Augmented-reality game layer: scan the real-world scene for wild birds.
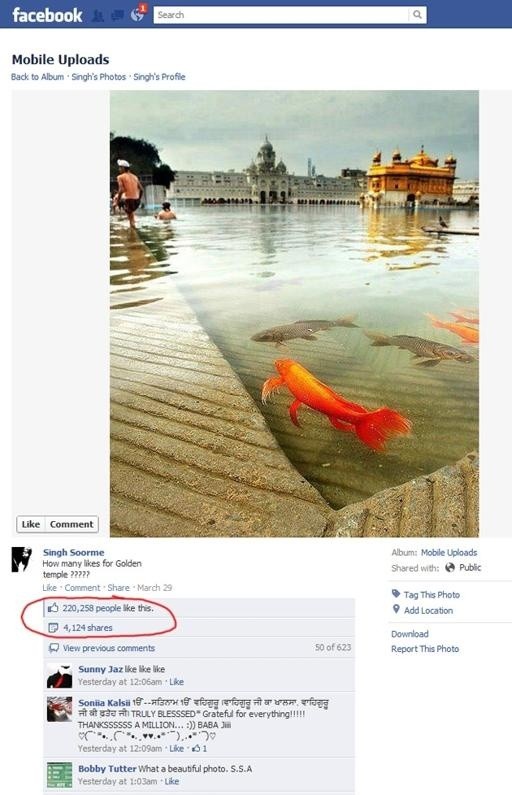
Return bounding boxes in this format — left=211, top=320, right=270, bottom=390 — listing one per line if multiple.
left=437, top=214, right=448, bottom=228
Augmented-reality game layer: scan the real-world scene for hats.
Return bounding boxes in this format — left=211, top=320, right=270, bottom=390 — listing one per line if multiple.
left=117, top=159, right=130, bottom=167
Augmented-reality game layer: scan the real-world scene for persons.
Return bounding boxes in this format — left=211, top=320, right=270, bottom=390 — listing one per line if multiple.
left=111, top=159, right=144, bottom=230
left=155, top=202, right=176, bottom=219
